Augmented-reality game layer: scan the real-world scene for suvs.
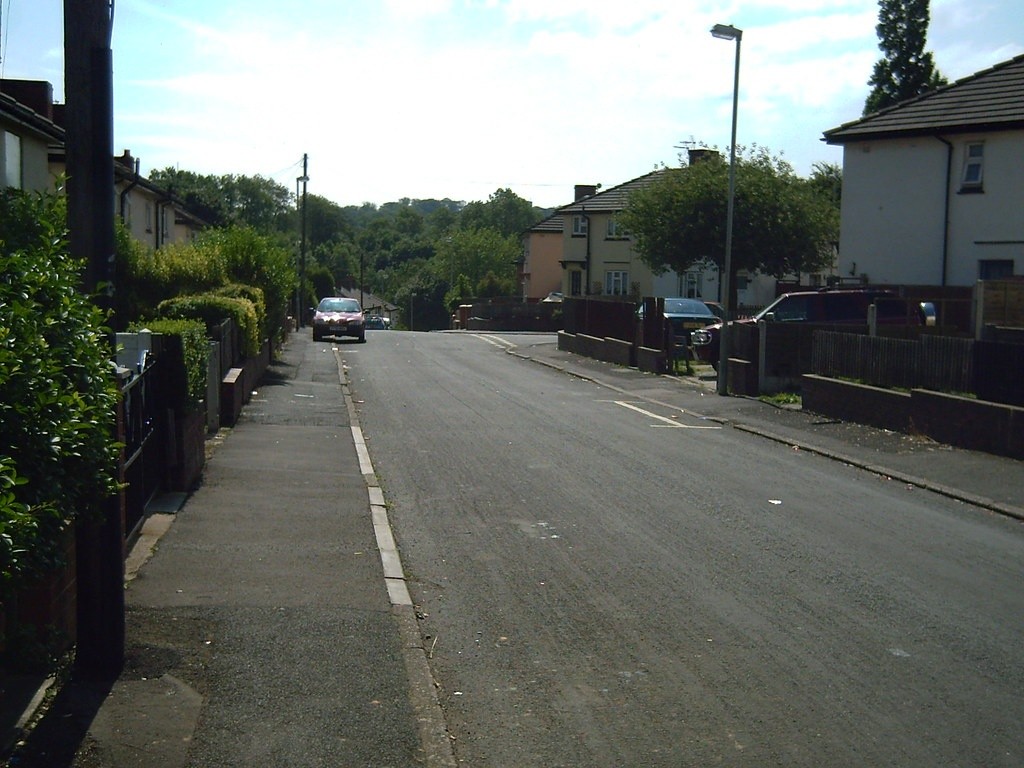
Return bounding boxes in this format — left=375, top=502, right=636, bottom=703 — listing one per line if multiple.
left=692, top=282, right=938, bottom=389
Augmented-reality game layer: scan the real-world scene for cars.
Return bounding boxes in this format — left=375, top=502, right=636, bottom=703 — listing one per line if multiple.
left=364, top=313, right=391, bottom=330
left=308, top=296, right=371, bottom=344
left=635, top=297, right=722, bottom=344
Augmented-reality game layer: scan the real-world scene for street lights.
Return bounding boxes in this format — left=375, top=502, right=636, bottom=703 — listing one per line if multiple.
left=295, top=175, right=310, bottom=333
left=708, top=22, right=745, bottom=395
